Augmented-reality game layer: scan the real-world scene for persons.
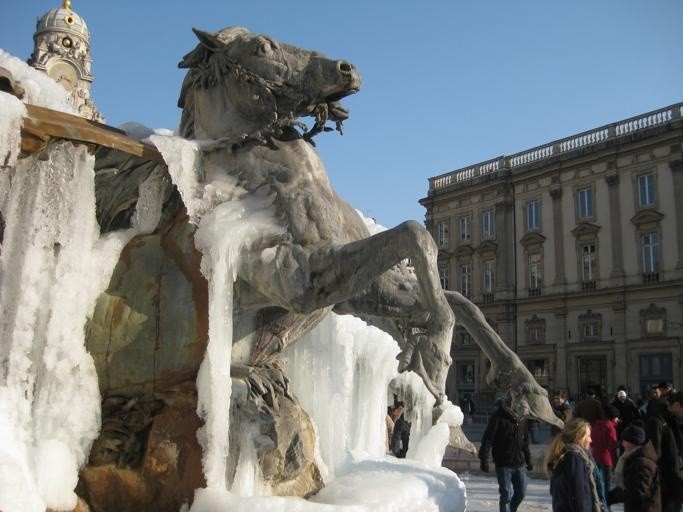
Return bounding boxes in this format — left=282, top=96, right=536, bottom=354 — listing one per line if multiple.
left=40, top=35, right=63, bottom=64
left=67, top=40, right=82, bottom=60
left=503, top=383, right=517, bottom=410
left=387, top=405, right=394, bottom=452
left=477, top=399, right=534, bottom=512
left=390, top=399, right=411, bottom=460
left=546, top=380, right=682, bottom=512
left=391, top=407, right=398, bottom=423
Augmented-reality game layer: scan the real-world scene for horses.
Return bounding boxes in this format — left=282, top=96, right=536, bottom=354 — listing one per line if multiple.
left=95, top=26, right=564, bottom=432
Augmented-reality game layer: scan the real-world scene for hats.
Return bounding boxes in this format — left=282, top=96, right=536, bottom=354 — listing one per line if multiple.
left=617, top=389, right=628, bottom=398
left=619, top=425, right=646, bottom=446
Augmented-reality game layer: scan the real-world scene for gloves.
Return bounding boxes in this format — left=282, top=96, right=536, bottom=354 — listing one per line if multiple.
left=480, top=460, right=489, bottom=473
left=527, top=460, right=533, bottom=471
